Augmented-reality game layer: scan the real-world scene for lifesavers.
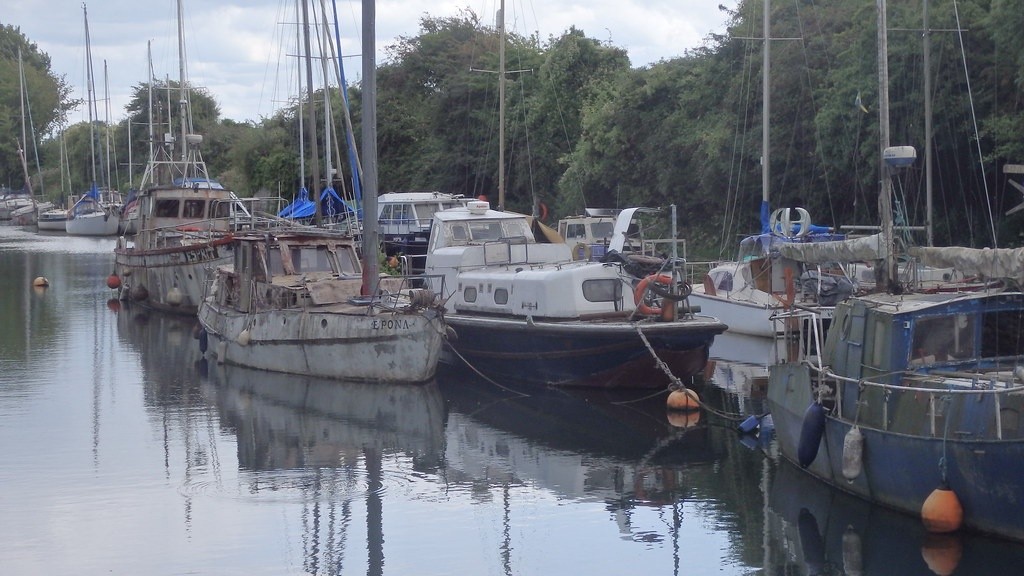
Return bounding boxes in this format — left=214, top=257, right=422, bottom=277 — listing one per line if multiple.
left=633, top=275, right=675, bottom=315
left=573, top=243, right=590, bottom=261
left=175, top=226, right=202, bottom=231
left=539, top=203, right=547, bottom=220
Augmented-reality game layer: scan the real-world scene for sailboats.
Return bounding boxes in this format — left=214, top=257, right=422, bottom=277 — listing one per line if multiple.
left=766, top=0, right=1024, bottom=543
left=0, top=0, right=941, bottom=385
